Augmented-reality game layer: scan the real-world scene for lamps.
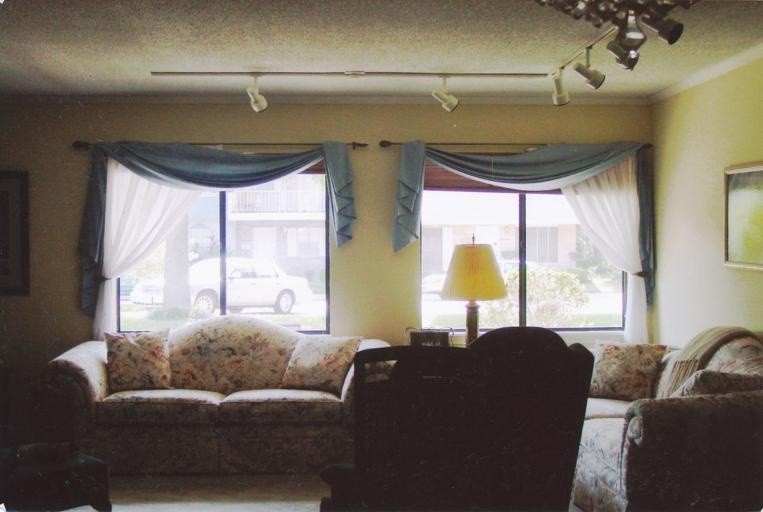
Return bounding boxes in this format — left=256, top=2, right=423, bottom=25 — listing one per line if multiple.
left=440, top=233, right=508, bottom=347
left=151, top=1, right=695, bottom=112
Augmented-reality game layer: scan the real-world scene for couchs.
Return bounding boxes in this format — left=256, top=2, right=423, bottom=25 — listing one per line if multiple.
left=42, top=314, right=394, bottom=478
left=574, top=325, right=763, bottom=512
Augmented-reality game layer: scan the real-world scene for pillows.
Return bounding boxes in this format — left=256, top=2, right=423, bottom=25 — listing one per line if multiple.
left=587, top=338, right=667, bottom=400
left=103, top=322, right=361, bottom=394
left=666, top=369, right=763, bottom=396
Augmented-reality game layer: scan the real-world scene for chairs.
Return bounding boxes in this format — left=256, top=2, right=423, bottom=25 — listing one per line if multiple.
left=319, top=328, right=594, bottom=512
left=0, top=362, right=114, bottom=512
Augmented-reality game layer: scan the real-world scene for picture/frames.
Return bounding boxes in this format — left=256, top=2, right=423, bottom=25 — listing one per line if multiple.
left=722, top=161, right=763, bottom=272
left=0, top=170, right=29, bottom=296
left=404, top=326, right=454, bottom=347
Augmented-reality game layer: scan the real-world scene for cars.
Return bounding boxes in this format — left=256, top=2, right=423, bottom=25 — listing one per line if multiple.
left=423, top=259, right=533, bottom=295
left=132, top=259, right=310, bottom=315
